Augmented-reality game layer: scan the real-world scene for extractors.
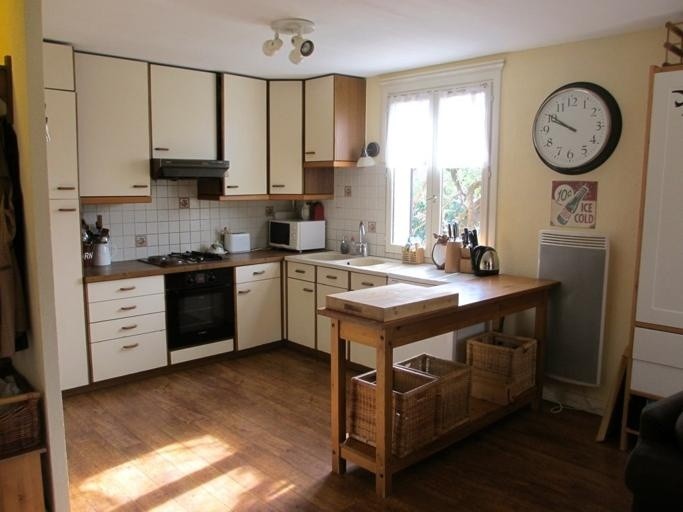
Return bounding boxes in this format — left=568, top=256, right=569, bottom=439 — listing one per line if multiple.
left=150, top=158, right=230, bottom=181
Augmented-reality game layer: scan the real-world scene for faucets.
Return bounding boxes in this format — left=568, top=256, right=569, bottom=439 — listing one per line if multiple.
left=357, top=220, right=366, bottom=255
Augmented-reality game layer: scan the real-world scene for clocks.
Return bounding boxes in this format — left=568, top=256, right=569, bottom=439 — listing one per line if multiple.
left=532, top=82, right=620, bottom=175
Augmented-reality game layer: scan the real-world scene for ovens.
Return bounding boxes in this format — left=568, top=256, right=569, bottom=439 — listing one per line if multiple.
left=162, top=266, right=233, bottom=351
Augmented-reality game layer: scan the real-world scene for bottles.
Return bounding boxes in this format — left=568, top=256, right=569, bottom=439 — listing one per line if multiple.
left=82, top=243, right=94, bottom=268
left=556, top=186, right=588, bottom=225
left=340, top=221, right=365, bottom=256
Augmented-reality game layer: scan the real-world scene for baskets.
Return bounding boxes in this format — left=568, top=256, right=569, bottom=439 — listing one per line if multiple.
left=349, top=367, right=435, bottom=458
left=466, top=331, right=537, bottom=406
left=1, top=359, right=41, bottom=457
left=393, top=353, right=469, bottom=432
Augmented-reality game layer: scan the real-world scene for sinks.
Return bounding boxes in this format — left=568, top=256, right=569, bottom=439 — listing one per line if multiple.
left=342, top=257, right=386, bottom=268
left=300, top=253, right=355, bottom=263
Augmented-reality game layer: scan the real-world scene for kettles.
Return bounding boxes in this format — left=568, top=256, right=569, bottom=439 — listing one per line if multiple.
left=468, top=244, right=500, bottom=277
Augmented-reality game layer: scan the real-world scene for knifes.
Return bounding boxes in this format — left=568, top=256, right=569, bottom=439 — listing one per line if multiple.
left=448, top=222, right=478, bottom=246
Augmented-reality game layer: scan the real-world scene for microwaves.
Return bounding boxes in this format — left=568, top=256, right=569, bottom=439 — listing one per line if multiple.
left=268, top=217, right=325, bottom=254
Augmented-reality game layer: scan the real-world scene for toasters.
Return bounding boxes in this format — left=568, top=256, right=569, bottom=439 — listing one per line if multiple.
left=223, top=231, right=250, bottom=253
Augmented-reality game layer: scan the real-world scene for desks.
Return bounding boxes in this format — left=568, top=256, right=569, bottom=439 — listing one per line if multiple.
left=316, top=272, right=564, bottom=500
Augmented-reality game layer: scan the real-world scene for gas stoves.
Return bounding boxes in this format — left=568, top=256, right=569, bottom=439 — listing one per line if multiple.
left=138, top=250, right=223, bottom=268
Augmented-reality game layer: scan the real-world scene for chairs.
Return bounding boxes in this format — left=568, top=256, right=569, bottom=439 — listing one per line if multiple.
left=622, top=393, right=682, bottom=512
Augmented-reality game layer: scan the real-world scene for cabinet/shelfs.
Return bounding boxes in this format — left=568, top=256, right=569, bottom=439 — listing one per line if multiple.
left=287, top=258, right=353, bottom=363
left=230, top=250, right=283, bottom=353
left=628, top=65, right=681, bottom=399
left=31, top=38, right=91, bottom=400
left=268, top=73, right=335, bottom=201
left=66, top=48, right=151, bottom=207
left=148, top=62, right=218, bottom=179
left=0, top=446, right=49, bottom=512
left=83, top=258, right=167, bottom=390
left=304, top=76, right=366, bottom=168
left=345, top=274, right=386, bottom=371
left=198, top=72, right=269, bottom=202
left=392, top=275, right=458, bottom=374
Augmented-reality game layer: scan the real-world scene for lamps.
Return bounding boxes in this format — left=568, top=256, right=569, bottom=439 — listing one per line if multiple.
left=356, top=142, right=380, bottom=167
left=262, top=18, right=316, bottom=65
